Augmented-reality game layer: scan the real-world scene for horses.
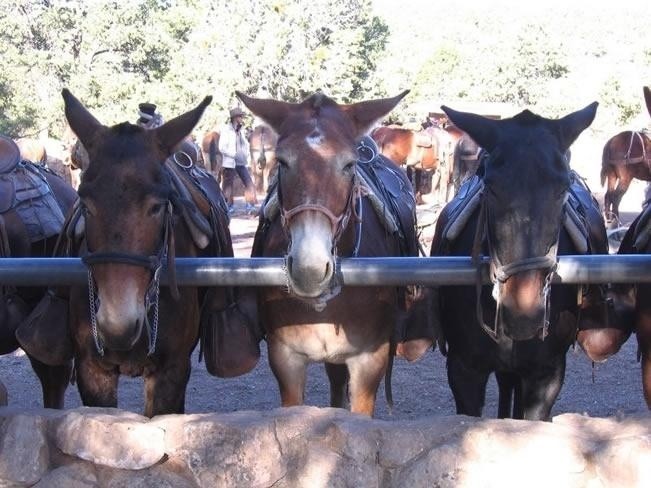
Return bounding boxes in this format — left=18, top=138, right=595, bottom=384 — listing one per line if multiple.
left=200, top=120, right=276, bottom=197
left=3, top=144, right=91, bottom=420
left=428, top=101, right=607, bottom=422
left=371, top=109, right=501, bottom=198
left=58, top=91, right=231, bottom=417
left=237, top=88, right=422, bottom=416
left=601, top=124, right=651, bottom=221
left=616, top=88, right=650, bottom=421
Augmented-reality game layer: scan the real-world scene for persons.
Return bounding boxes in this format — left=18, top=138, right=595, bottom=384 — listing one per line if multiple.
left=373, top=113, right=461, bottom=211
left=215, top=105, right=261, bottom=216
